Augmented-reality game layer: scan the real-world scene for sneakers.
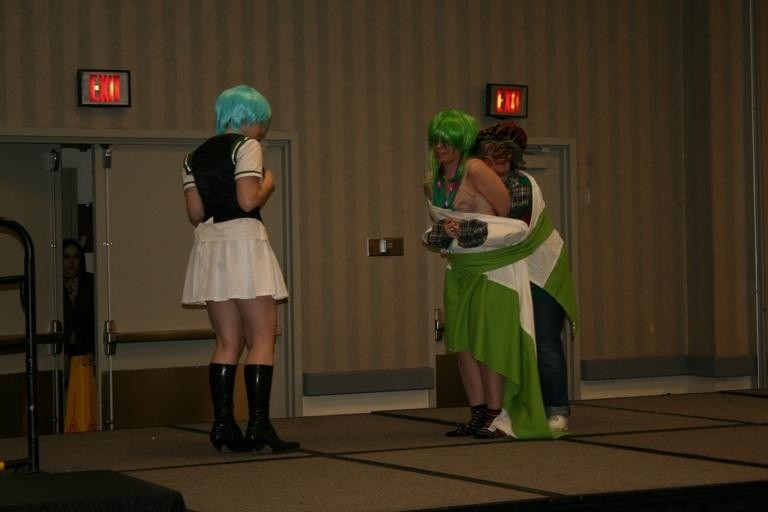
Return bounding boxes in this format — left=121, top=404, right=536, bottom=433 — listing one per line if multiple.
left=546, top=415, right=569, bottom=432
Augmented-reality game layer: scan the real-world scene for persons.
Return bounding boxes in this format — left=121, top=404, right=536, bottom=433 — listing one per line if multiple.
left=421, top=120, right=580, bottom=436
left=60, top=236, right=96, bottom=433
left=421, top=108, right=513, bottom=440
left=182, top=84, right=303, bottom=452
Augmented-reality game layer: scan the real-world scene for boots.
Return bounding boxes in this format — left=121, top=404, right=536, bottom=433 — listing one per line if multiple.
left=210, top=364, right=253, bottom=454
left=245, top=364, right=299, bottom=454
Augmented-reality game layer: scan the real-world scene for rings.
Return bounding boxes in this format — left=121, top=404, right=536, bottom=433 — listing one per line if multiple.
left=450, top=227, right=455, bottom=232
